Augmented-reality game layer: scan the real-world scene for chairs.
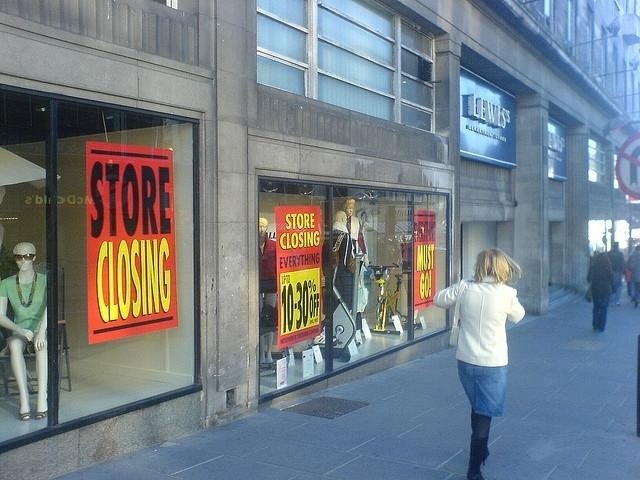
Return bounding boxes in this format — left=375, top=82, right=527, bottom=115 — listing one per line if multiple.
left=0, top=269, right=71, bottom=393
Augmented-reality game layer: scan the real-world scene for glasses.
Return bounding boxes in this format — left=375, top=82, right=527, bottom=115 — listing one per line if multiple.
left=14, top=253, right=36, bottom=262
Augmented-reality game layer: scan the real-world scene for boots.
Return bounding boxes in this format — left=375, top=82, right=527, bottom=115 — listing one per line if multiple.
left=466, top=434, right=489, bottom=480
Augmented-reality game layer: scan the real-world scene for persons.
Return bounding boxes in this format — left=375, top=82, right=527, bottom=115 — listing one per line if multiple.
left=0, top=241, right=49, bottom=422
left=432, top=246, right=528, bottom=480
left=624, top=237, right=636, bottom=261
left=334, top=211, right=359, bottom=360
left=344, top=197, right=368, bottom=347
left=589, top=241, right=615, bottom=333
left=258, top=217, right=275, bottom=366
left=608, top=242, right=624, bottom=307
left=627, top=244, right=640, bottom=308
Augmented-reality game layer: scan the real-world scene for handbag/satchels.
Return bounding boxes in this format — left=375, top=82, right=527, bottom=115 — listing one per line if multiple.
left=450, top=280, right=467, bottom=348
left=332, top=232, right=344, bottom=267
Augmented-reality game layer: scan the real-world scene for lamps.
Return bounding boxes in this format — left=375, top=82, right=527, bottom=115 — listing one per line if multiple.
left=592, top=55, right=640, bottom=84
left=565, top=14, right=621, bottom=55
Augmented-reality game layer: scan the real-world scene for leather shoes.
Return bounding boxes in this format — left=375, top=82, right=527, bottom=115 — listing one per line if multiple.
left=34, top=404, right=48, bottom=419
left=19, top=409, right=31, bottom=420
